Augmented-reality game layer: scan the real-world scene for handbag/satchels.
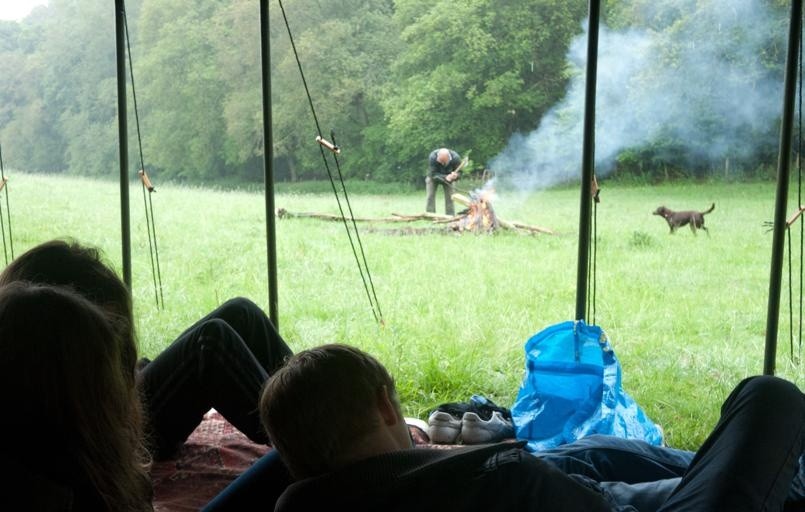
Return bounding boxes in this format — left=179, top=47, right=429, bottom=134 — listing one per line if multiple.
left=509, top=320, right=665, bottom=454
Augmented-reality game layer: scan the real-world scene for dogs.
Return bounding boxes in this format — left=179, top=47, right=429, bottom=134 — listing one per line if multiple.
left=652, top=202, right=716, bottom=239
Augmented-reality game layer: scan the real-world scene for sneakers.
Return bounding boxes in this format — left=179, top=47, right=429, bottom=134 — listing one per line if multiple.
left=428, top=410, right=461, bottom=443
left=462, top=411, right=515, bottom=444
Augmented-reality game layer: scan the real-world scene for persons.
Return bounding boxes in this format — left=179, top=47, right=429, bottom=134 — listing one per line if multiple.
left=1, top=284, right=154, bottom=512
left=0, top=236, right=138, bottom=404
left=262, top=337, right=804, bottom=511
left=423, top=147, right=466, bottom=215
left=139, top=292, right=296, bottom=449
